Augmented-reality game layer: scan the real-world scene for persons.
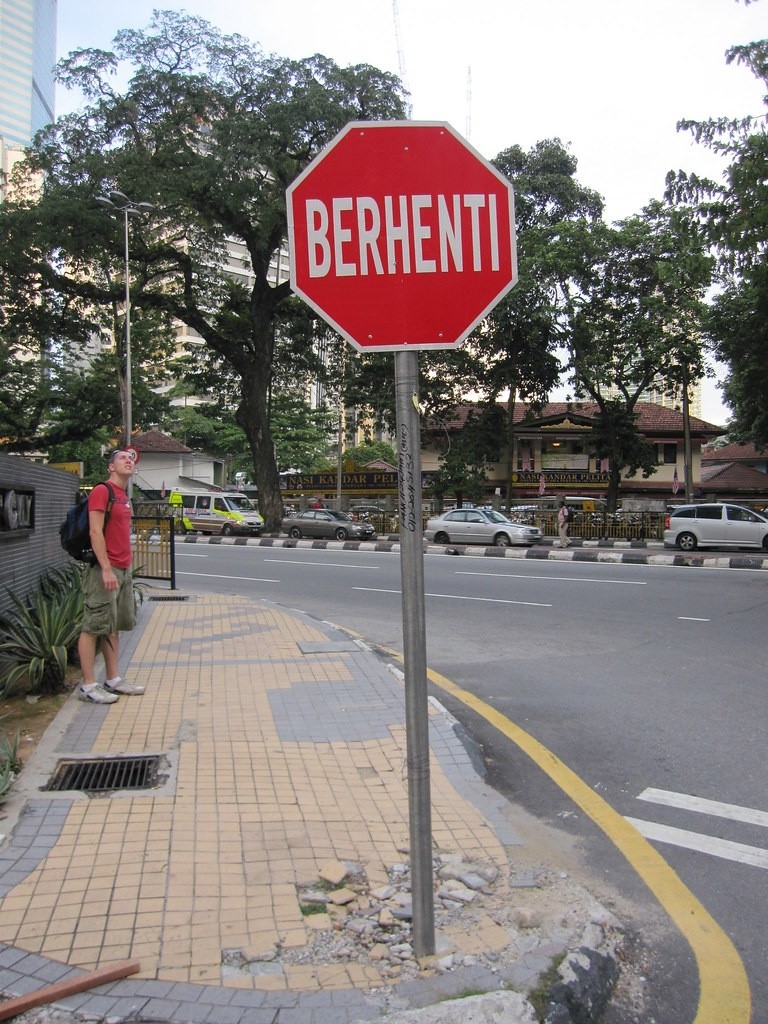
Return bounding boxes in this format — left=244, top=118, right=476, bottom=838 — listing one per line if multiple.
left=76, top=450, right=145, bottom=704
left=140, top=506, right=168, bottom=515
left=389, top=515, right=399, bottom=532
left=760, top=503, right=768, bottom=512
left=314, top=499, right=324, bottom=509
left=558, top=501, right=572, bottom=548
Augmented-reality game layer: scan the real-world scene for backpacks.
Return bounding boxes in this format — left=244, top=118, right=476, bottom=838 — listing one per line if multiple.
left=59, top=481, right=116, bottom=568
left=563, top=507, right=574, bottom=522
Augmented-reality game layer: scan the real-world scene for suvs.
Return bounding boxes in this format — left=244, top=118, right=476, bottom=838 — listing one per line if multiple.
left=662, top=502, right=768, bottom=552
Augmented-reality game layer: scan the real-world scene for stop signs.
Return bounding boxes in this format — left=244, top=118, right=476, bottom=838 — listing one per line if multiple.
left=285, top=118, right=519, bottom=353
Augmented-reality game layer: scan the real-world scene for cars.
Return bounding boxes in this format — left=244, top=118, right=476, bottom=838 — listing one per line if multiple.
left=442, top=501, right=539, bottom=525
left=346, top=506, right=386, bottom=524
left=424, top=508, right=543, bottom=547
left=280, top=508, right=377, bottom=542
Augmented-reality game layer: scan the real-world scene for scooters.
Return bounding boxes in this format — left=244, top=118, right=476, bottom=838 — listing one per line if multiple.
left=584, top=506, right=650, bottom=542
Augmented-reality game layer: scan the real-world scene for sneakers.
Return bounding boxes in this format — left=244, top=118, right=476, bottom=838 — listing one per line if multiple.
left=78, top=684, right=119, bottom=704
left=103, top=679, right=145, bottom=695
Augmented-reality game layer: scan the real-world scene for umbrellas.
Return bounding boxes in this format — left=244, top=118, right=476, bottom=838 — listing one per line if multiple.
left=161, top=483, right=165, bottom=497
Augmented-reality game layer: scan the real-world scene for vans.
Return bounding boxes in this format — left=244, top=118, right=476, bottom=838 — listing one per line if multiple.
left=537, top=496, right=606, bottom=511
left=166, top=486, right=266, bottom=537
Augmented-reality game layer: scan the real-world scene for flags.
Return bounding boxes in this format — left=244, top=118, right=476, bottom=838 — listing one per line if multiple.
left=673, top=469, right=680, bottom=494
left=540, top=475, right=545, bottom=495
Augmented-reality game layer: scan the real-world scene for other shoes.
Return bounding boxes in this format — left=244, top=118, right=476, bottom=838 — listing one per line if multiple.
left=566, top=541, right=573, bottom=547
left=557, top=546, right=567, bottom=549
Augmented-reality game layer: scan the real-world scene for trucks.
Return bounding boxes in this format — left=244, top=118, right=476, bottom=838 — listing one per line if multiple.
left=614, top=498, right=664, bottom=524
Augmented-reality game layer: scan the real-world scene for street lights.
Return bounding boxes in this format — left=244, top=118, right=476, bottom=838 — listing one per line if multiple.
left=93, top=189, right=157, bottom=514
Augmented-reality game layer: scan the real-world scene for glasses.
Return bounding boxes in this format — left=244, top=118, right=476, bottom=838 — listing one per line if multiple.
left=111, top=450, right=120, bottom=459
